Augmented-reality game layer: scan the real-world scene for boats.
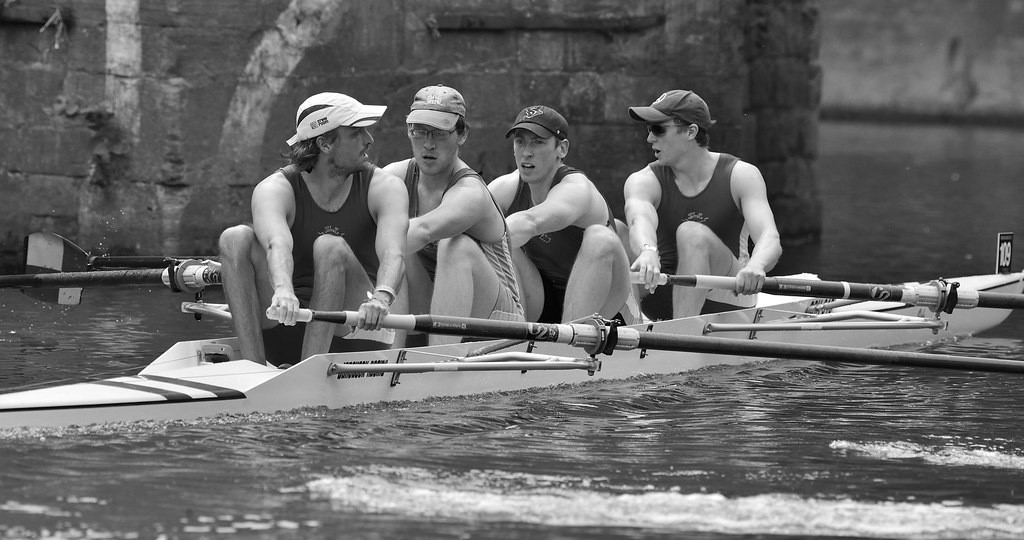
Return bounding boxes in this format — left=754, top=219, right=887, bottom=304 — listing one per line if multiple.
left=1, top=230, right=1024, bottom=435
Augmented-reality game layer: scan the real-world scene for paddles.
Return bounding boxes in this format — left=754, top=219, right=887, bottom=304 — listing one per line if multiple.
left=265, top=303, right=1024, bottom=374
left=628, top=269, right=1024, bottom=309
left=0, top=259, right=224, bottom=293
left=18, top=230, right=221, bottom=307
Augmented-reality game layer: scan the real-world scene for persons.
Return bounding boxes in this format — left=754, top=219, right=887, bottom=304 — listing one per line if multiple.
left=219, top=92, right=409, bottom=371
left=614, top=90, right=783, bottom=321
left=486, top=105, right=642, bottom=326
left=380, top=84, right=526, bottom=347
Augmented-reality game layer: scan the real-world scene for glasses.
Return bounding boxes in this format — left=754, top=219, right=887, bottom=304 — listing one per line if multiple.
left=409, top=128, right=458, bottom=139
left=647, top=124, right=690, bottom=136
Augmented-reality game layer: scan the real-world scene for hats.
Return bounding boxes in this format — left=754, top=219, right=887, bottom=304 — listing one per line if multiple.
left=505, top=105, right=569, bottom=140
left=406, top=84, right=466, bottom=130
left=628, top=90, right=711, bottom=129
left=287, top=91, right=388, bottom=146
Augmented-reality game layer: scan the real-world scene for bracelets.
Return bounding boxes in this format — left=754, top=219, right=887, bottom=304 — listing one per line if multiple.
left=364, top=285, right=397, bottom=310
left=640, top=245, right=660, bottom=253
